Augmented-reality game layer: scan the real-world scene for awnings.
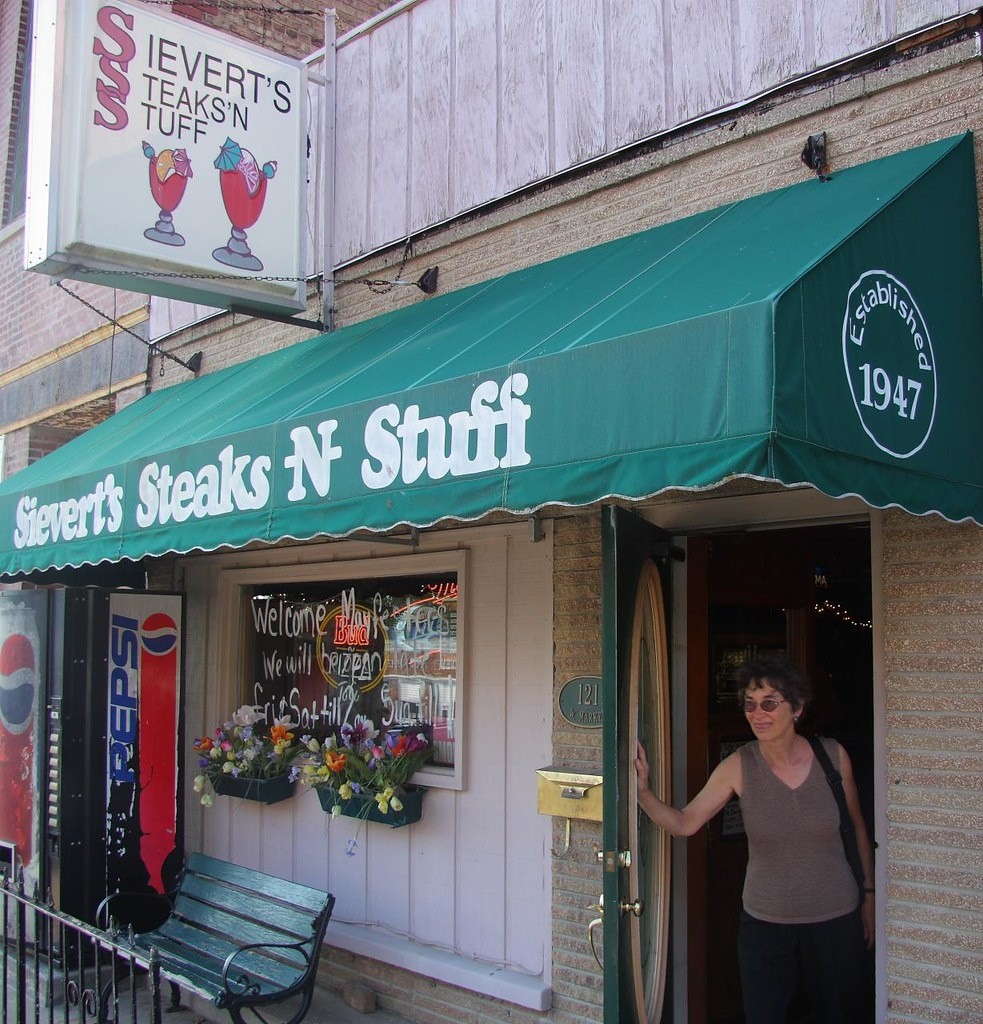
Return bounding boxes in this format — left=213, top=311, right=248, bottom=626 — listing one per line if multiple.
left=1, top=127, right=983, bottom=595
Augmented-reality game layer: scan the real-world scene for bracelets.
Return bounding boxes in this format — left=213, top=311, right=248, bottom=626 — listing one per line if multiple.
left=864, top=888, right=875, bottom=894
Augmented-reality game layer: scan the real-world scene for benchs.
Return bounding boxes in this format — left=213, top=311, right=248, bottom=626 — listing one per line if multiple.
left=96, top=852, right=336, bottom=1024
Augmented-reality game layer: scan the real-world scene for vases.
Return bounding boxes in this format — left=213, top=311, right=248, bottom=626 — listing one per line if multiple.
left=207, top=766, right=294, bottom=804
left=311, top=782, right=428, bottom=827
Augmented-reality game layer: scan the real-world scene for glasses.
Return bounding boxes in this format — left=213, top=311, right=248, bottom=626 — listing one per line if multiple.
left=739, top=699, right=790, bottom=712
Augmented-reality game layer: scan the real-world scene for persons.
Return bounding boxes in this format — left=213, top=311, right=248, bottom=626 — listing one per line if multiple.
left=635, top=647, right=878, bottom=1024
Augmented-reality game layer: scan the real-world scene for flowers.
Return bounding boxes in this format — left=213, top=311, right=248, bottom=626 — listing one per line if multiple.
left=193, top=705, right=294, bottom=807
left=287, top=716, right=429, bottom=858
